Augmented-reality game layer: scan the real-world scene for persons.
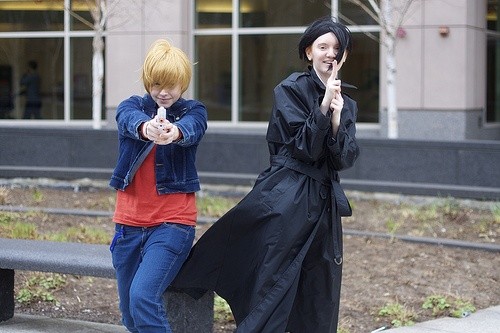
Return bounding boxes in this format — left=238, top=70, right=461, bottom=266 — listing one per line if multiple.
left=108, top=39, right=209, bottom=332
left=233, top=15, right=362, bottom=333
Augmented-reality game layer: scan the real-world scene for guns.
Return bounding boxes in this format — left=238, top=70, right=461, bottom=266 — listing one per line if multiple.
left=155, top=105, right=168, bottom=136
